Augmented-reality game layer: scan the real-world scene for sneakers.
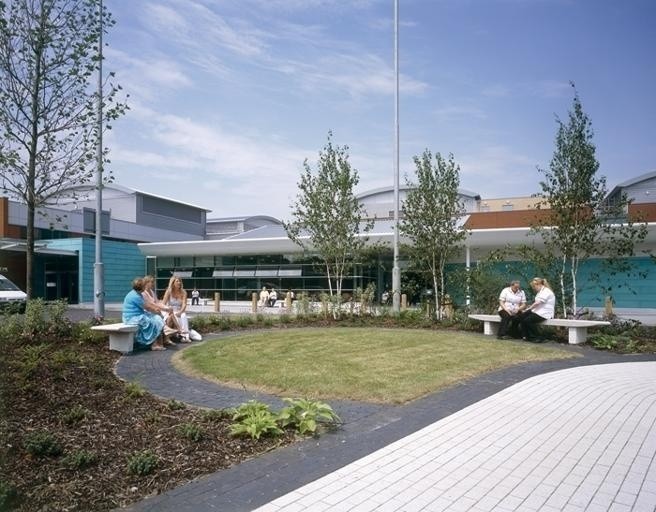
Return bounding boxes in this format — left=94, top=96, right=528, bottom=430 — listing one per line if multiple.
left=152, top=327, right=178, bottom=351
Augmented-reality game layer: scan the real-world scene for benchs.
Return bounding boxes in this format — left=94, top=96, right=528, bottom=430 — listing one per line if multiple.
left=91, top=323, right=138, bottom=352
left=468, top=313, right=610, bottom=344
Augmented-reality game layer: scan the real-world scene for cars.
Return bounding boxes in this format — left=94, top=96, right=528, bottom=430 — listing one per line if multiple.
left=379, top=282, right=432, bottom=305
left=237, top=282, right=289, bottom=300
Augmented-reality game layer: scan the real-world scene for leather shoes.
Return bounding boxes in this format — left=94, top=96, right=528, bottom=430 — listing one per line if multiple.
left=498, top=333, right=537, bottom=340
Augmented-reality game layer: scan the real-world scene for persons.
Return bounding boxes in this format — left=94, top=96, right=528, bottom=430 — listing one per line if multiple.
left=258, top=287, right=277, bottom=307
left=287, top=288, right=294, bottom=305
left=122, top=275, right=202, bottom=351
left=497, top=277, right=556, bottom=343
left=382, top=290, right=389, bottom=304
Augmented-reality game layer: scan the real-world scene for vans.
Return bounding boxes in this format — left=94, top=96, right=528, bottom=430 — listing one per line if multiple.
left=0, top=273, right=28, bottom=315
